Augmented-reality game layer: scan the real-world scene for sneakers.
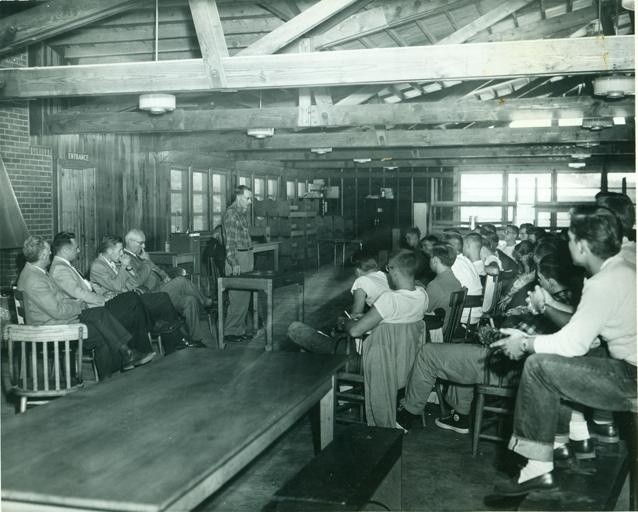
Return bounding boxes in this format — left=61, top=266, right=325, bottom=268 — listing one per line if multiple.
left=433, top=409, right=471, bottom=436
left=388, top=405, right=421, bottom=435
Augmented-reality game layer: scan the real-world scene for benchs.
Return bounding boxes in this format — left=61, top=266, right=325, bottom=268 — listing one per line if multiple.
left=260, top=425, right=404, bottom=512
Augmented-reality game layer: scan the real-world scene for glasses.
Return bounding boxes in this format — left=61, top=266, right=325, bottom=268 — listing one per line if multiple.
left=129, top=238, right=146, bottom=246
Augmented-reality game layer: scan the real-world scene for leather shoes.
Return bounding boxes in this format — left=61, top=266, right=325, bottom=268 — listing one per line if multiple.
left=120, top=348, right=158, bottom=371
left=545, top=421, right=622, bottom=470
left=174, top=337, right=202, bottom=350
left=222, top=332, right=253, bottom=343
left=493, top=468, right=562, bottom=498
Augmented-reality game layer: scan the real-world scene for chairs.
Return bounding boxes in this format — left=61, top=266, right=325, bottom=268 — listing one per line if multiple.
left=332, top=268, right=526, bottom=456
left=3, top=322, right=88, bottom=414
left=13, top=286, right=99, bottom=384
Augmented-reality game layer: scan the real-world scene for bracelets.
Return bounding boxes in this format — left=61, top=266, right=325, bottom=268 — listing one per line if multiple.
left=127, top=266, right=133, bottom=272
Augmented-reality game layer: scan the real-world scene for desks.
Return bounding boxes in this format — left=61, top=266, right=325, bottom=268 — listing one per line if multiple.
left=253, top=241, right=283, bottom=271
left=1, top=346, right=356, bottom=512
left=147, top=251, right=196, bottom=283
left=217, top=269, right=304, bottom=353
left=316, top=238, right=363, bottom=272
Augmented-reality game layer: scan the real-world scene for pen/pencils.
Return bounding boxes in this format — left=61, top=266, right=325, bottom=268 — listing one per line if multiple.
left=344, top=310, right=352, bottom=320
left=489, top=317, right=496, bottom=332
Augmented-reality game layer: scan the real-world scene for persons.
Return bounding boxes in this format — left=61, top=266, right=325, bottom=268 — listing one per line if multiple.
left=287, top=191, right=637, bottom=498
left=224, top=184, right=256, bottom=343
left=204, top=225, right=227, bottom=278
left=18, top=233, right=158, bottom=382
left=48, top=232, right=154, bottom=355
left=90, top=235, right=203, bottom=355
left=123, top=231, right=218, bottom=348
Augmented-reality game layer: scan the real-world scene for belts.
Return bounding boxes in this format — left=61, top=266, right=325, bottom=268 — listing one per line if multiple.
left=235, top=246, right=255, bottom=253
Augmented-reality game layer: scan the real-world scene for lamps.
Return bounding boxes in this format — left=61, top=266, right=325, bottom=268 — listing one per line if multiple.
left=568, top=0, right=635, bottom=168
left=138, top=0, right=176, bottom=116
left=383, top=166, right=399, bottom=170
left=246, top=89, right=275, bottom=139
left=311, top=128, right=333, bottom=155
left=353, top=158, right=371, bottom=164
